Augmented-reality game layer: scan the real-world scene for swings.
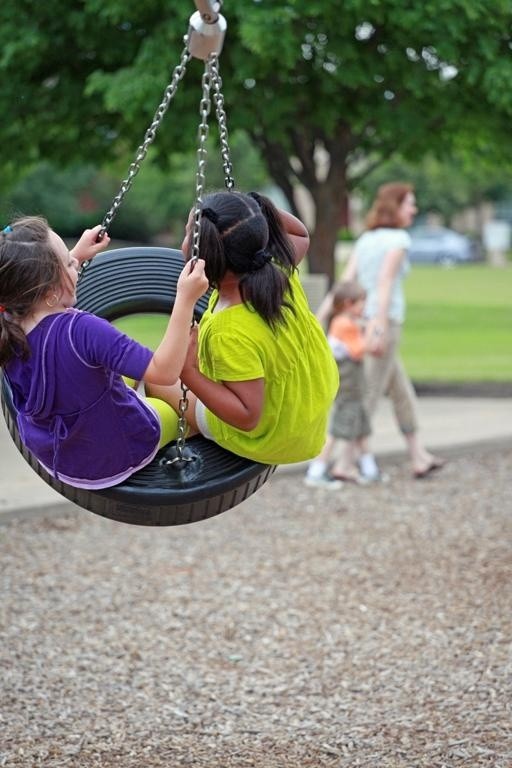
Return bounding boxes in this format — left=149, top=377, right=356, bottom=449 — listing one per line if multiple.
left=0, top=2, right=277, bottom=525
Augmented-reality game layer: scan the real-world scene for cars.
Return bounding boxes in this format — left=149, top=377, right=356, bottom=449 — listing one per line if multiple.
left=406, top=223, right=484, bottom=268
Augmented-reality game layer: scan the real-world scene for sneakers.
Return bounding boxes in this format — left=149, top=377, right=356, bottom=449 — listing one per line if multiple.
left=302, top=462, right=394, bottom=491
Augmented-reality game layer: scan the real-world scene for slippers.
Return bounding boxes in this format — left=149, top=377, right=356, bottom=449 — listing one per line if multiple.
left=412, top=452, right=450, bottom=481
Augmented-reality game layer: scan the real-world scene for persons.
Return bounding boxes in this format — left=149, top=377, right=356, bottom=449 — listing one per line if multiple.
left=139, top=188, right=340, bottom=465
left=303, top=280, right=380, bottom=491
left=312, top=181, right=453, bottom=489
left=0, top=212, right=213, bottom=499
left=479, top=209, right=511, bottom=266
